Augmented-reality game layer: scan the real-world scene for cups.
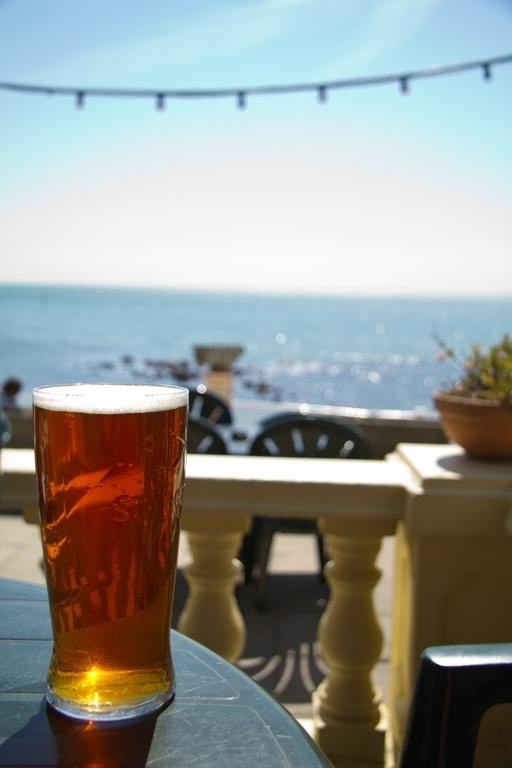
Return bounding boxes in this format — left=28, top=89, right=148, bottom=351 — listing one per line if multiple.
left=30, top=378, right=193, bottom=730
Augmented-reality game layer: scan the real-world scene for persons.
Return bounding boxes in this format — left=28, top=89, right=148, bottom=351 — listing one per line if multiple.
left=0, top=376, right=23, bottom=407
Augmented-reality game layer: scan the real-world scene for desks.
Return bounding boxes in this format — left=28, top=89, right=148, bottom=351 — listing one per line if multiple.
left=0, top=577, right=343, bottom=767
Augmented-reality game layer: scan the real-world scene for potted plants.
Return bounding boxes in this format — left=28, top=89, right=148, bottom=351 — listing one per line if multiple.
left=431, top=328, right=512, bottom=463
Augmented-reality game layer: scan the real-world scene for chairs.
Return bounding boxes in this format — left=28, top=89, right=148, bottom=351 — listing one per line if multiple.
left=188, top=385, right=374, bottom=582
